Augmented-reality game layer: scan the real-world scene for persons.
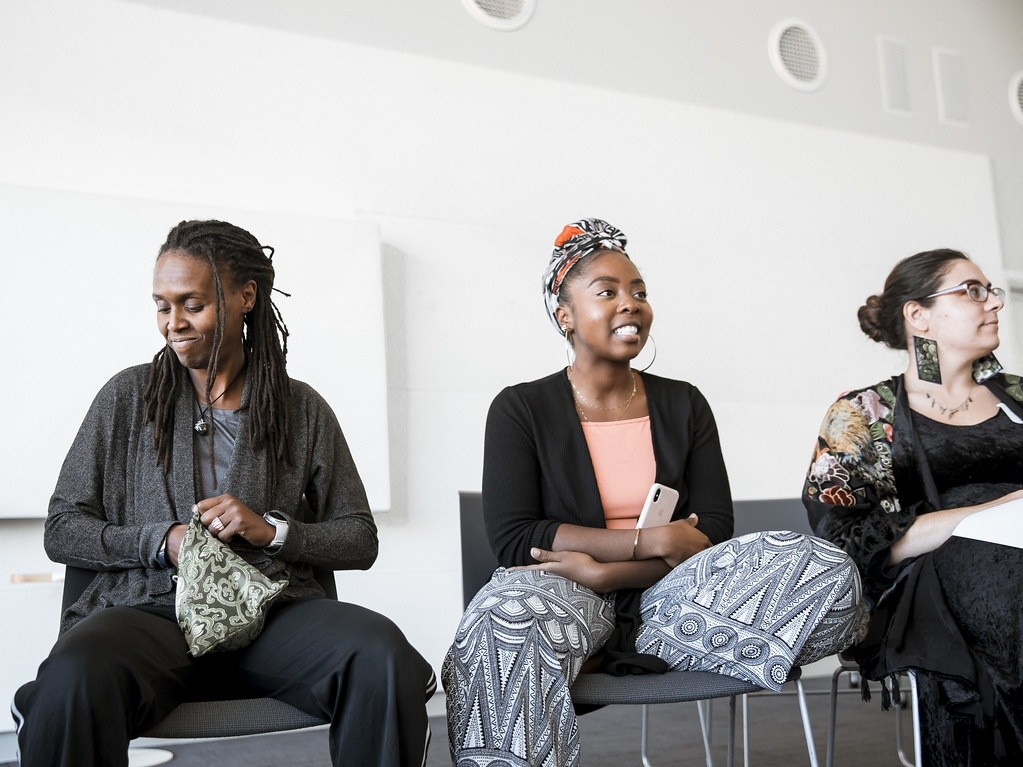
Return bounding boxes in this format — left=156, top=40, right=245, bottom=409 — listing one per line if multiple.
left=801, top=248, right=1023, bottom=767
left=439, top=217, right=872, bottom=767
left=9, top=219, right=437, bottom=767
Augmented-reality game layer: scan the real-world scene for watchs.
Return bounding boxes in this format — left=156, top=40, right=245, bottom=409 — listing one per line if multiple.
left=263, top=511, right=289, bottom=548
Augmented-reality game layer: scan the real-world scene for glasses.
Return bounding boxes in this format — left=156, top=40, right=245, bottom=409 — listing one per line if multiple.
left=920, top=283, right=1006, bottom=303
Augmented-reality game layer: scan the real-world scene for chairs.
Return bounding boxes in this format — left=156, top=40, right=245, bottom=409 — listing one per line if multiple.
left=707, top=499, right=816, bottom=766
left=61, top=558, right=337, bottom=738
left=827, top=651, right=922, bottom=767
left=459, top=492, right=817, bottom=767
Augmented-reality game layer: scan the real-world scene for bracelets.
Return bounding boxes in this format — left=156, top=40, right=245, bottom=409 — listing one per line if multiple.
left=156, top=536, right=168, bottom=567
left=632, top=528, right=641, bottom=561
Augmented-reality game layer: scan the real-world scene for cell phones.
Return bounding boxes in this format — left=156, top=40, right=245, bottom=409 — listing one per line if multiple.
left=635, top=483, right=679, bottom=530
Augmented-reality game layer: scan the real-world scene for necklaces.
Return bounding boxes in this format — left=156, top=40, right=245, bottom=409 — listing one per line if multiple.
left=189, top=359, right=246, bottom=435
left=567, top=366, right=637, bottom=422
left=907, top=376, right=974, bottom=419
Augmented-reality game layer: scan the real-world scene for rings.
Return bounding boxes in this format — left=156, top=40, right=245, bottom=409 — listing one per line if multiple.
left=212, top=517, right=224, bottom=530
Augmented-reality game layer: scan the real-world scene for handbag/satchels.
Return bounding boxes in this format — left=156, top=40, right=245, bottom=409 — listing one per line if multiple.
left=171, top=513, right=290, bottom=658
